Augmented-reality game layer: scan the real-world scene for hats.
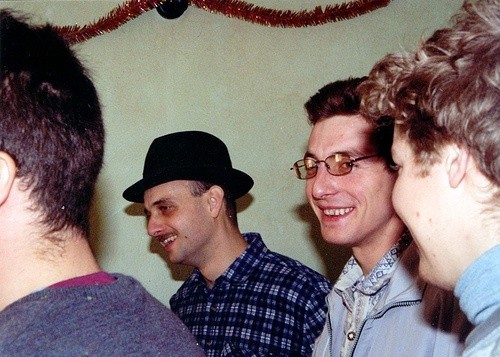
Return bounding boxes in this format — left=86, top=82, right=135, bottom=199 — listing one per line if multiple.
left=122, top=130, right=255, bottom=204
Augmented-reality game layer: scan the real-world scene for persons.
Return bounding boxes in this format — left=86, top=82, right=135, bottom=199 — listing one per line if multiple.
left=122, top=132, right=334, bottom=357
left=356, top=1, right=500, bottom=357
left=1, top=6, right=206, bottom=357
left=290, top=77, right=475, bottom=357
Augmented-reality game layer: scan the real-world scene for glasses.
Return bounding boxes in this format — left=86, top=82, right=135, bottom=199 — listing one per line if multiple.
left=289, top=151, right=388, bottom=180
